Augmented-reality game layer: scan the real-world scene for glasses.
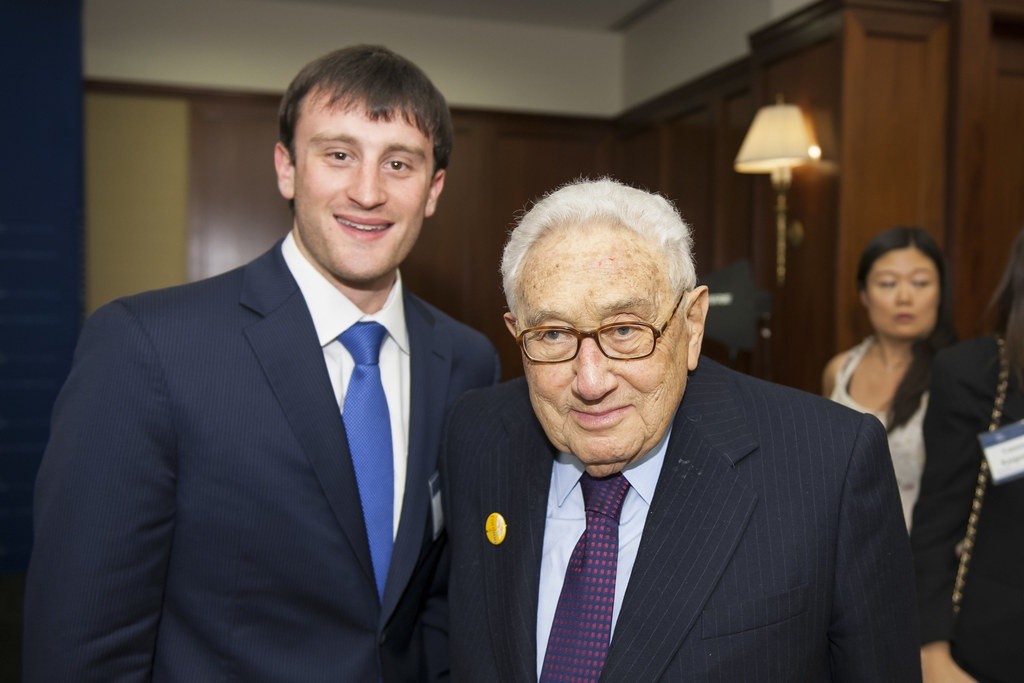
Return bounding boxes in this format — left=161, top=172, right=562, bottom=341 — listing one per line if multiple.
left=514, top=289, right=688, bottom=364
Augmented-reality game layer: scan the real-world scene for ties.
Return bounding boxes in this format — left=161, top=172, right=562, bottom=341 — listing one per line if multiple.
left=338, top=320, right=396, bottom=603
left=537, top=470, right=629, bottom=683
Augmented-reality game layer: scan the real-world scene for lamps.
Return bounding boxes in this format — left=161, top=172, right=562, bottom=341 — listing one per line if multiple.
left=734, top=93, right=807, bottom=283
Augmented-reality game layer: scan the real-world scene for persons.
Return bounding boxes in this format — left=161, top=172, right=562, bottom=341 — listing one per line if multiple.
left=23, top=46, right=503, bottom=683
left=827, top=224, right=962, bottom=532
left=911, top=230, right=1024, bottom=682
left=440, top=171, right=923, bottom=683
